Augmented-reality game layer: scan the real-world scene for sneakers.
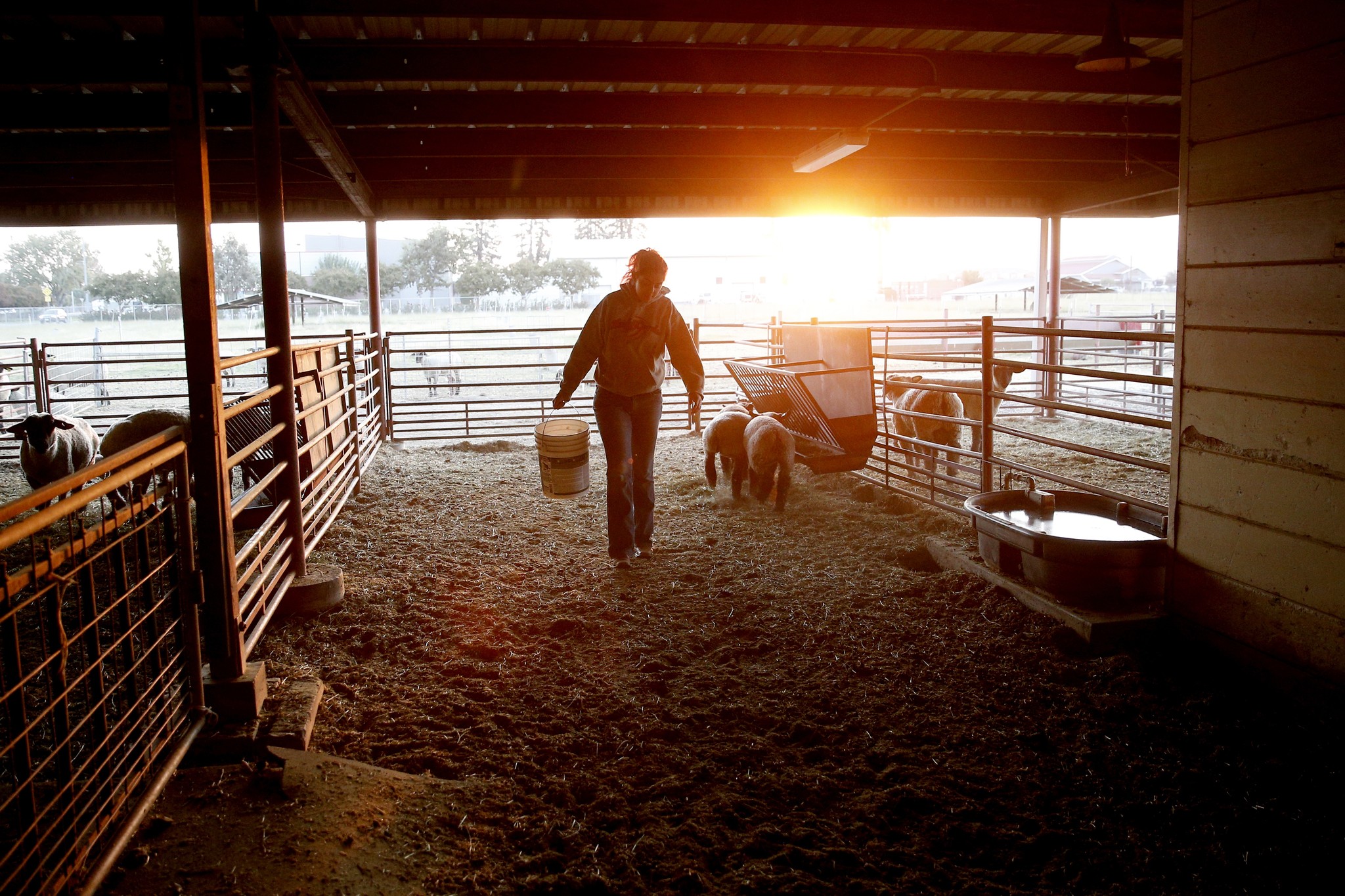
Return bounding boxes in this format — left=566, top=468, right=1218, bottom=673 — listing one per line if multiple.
left=633, top=545, right=653, bottom=558
left=612, top=554, right=631, bottom=568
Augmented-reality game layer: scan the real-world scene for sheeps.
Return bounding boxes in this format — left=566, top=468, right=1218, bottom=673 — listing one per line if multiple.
left=701, top=400, right=796, bottom=512
left=887, top=363, right=1025, bottom=453
left=4, top=412, right=100, bottom=516
left=410, top=352, right=462, bottom=397
left=101, top=402, right=271, bottom=521
left=880, top=375, right=965, bottom=485
left=0, top=362, right=73, bottom=433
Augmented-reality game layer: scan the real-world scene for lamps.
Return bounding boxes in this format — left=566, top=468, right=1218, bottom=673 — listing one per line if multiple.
left=791, top=128, right=871, bottom=175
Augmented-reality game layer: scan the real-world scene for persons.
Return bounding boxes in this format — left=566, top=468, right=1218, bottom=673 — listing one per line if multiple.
left=553, top=250, right=704, bottom=567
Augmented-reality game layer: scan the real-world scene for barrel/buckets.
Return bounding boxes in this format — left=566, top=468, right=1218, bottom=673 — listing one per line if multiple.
left=533, top=401, right=591, bottom=500
